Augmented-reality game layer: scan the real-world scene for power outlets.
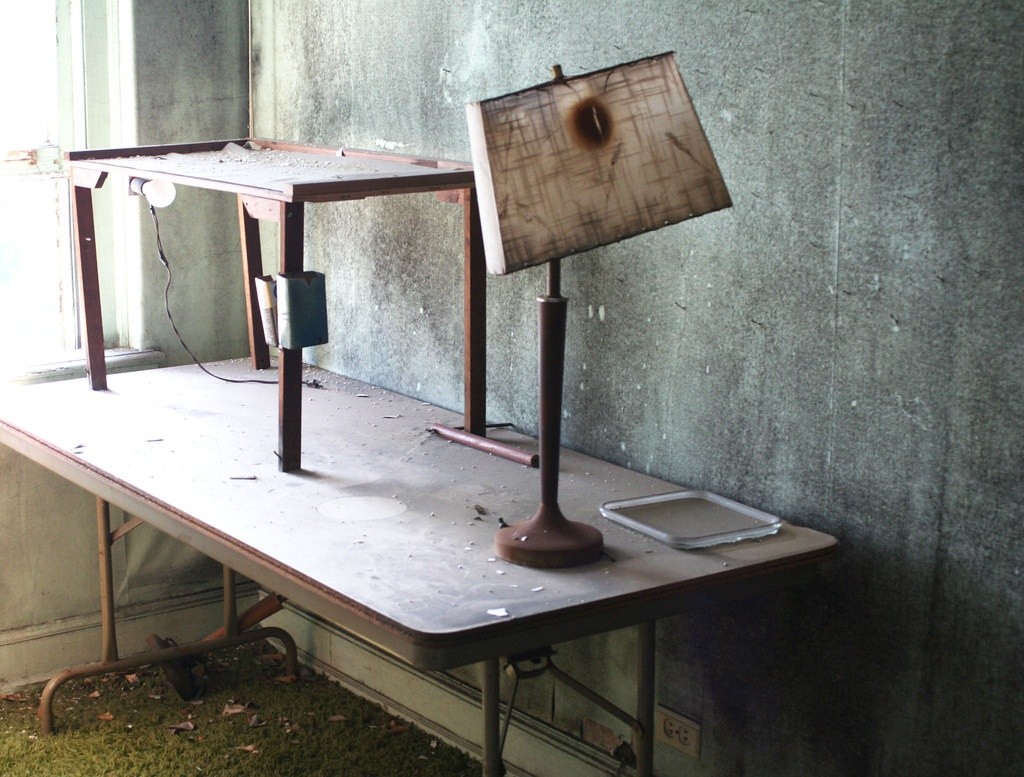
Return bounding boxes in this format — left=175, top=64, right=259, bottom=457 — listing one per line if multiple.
left=656, top=704, right=701, bottom=761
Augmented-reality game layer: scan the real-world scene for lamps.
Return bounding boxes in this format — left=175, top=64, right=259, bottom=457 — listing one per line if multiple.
left=462, top=48, right=734, bottom=573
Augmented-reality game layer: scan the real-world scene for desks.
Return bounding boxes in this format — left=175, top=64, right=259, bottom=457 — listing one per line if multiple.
left=62, top=137, right=481, bottom=474
left=0, top=355, right=839, bottom=777
